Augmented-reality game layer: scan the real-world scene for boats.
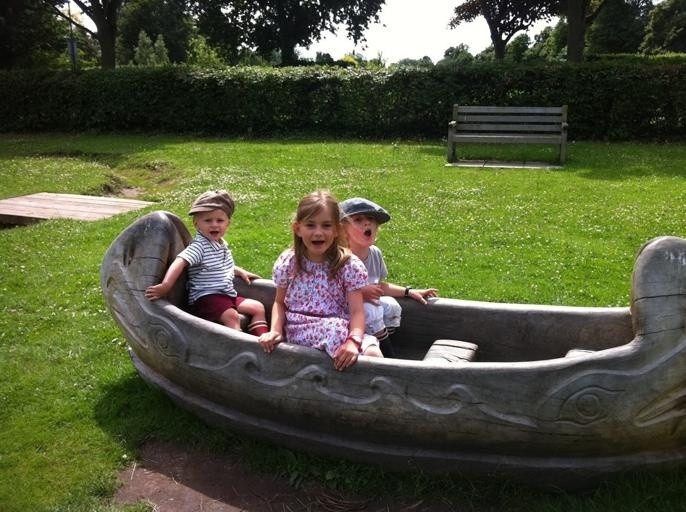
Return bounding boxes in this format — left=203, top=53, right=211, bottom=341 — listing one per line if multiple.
left=97, top=209, right=686, bottom=479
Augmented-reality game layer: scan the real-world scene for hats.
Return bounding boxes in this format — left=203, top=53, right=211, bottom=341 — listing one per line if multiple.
left=338, top=196, right=391, bottom=225
left=187, top=189, right=235, bottom=218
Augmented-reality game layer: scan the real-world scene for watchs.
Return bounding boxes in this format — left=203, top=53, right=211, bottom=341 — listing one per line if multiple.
left=405, top=285, right=413, bottom=297
left=344, top=334, right=364, bottom=353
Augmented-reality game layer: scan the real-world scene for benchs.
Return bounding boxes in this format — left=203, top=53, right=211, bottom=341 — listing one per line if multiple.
left=446, top=104, right=570, bottom=166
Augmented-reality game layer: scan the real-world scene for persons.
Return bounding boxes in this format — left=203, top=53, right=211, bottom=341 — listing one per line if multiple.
left=336, top=197, right=441, bottom=358
left=144, top=189, right=269, bottom=338
left=258, top=190, right=386, bottom=372
